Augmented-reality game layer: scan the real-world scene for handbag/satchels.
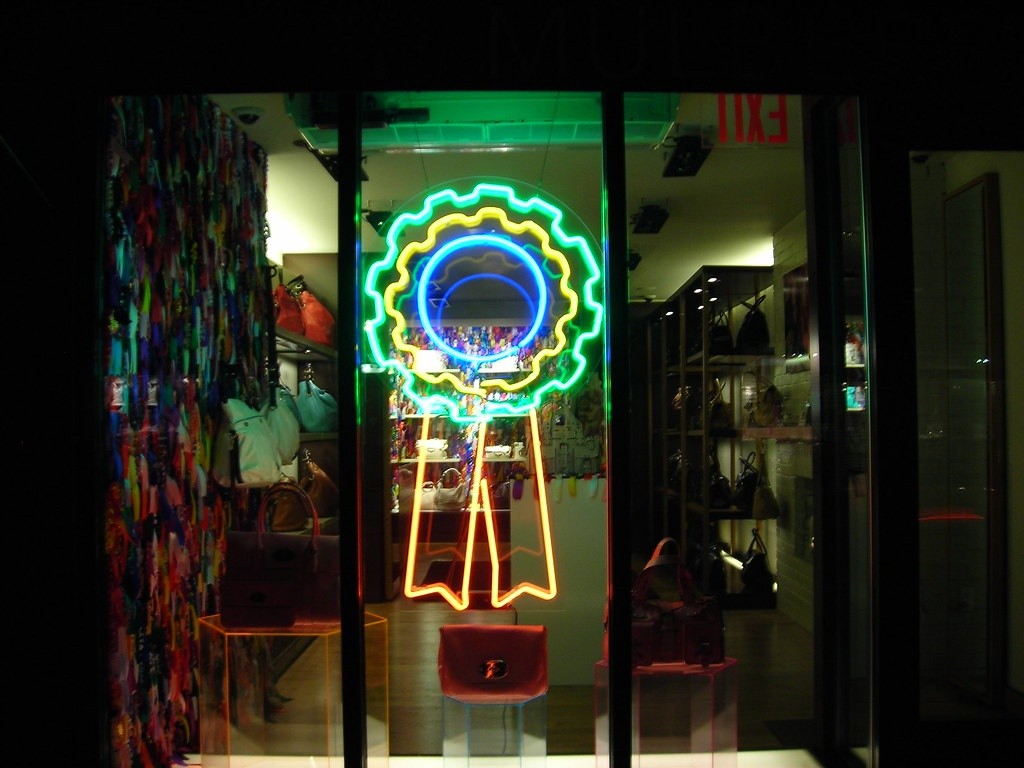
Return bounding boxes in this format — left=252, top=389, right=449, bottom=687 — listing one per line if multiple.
left=705, top=298, right=734, bottom=355
left=621, top=552, right=688, bottom=676
left=622, top=536, right=732, bottom=662
left=667, top=384, right=701, bottom=428
left=485, top=445, right=512, bottom=460
left=417, top=437, right=448, bottom=460
left=437, top=624, right=549, bottom=705
left=218, top=484, right=346, bottom=630
left=734, top=452, right=759, bottom=510
left=706, top=378, right=734, bottom=436
left=708, top=442, right=734, bottom=509
left=288, top=363, right=347, bottom=436
left=513, top=441, right=525, bottom=461
left=667, top=447, right=694, bottom=492
left=493, top=480, right=513, bottom=508
left=740, top=528, right=774, bottom=591
left=735, top=294, right=774, bottom=352
left=693, top=543, right=726, bottom=595
left=271, top=278, right=301, bottom=334
left=242, top=374, right=304, bottom=462
left=433, top=467, right=463, bottom=510
left=270, top=477, right=310, bottom=531
left=292, top=275, right=337, bottom=343
left=421, top=480, right=436, bottom=510
left=211, top=373, right=284, bottom=490
left=752, top=452, right=782, bottom=521
left=300, top=450, right=342, bottom=515
left=744, top=370, right=786, bottom=428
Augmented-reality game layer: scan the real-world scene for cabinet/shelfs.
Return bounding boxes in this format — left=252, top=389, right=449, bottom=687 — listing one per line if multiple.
left=647, top=262, right=778, bottom=612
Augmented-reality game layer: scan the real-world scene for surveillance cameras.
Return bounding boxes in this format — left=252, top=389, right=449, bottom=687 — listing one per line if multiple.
left=231, top=107, right=263, bottom=125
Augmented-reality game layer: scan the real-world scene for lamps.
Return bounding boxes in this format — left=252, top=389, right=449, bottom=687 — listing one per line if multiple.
left=634, top=205, right=670, bottom=235
left=662, top=134, right=715, bottom=176
left=366, top=210, right=405, bottom=237
left=302, top=142, right=369, bottom=184
left=627, top=250, right=642, bottom=270
